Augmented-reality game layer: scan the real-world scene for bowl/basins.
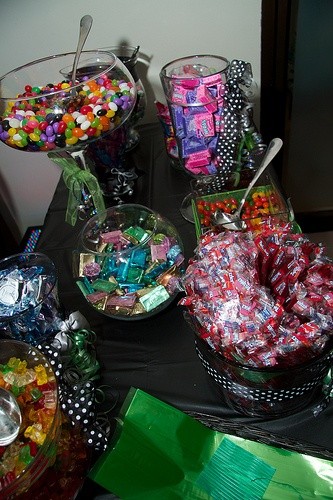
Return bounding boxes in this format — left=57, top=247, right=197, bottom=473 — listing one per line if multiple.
left=0, top=339, right=62, bottom=500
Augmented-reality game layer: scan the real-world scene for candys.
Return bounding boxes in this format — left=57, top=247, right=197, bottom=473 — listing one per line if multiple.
left=154, top=64, right=263, bottom=179
left=0, top=70, right=136, bottom=151
left=0, top=189, right=333, bottom=500
